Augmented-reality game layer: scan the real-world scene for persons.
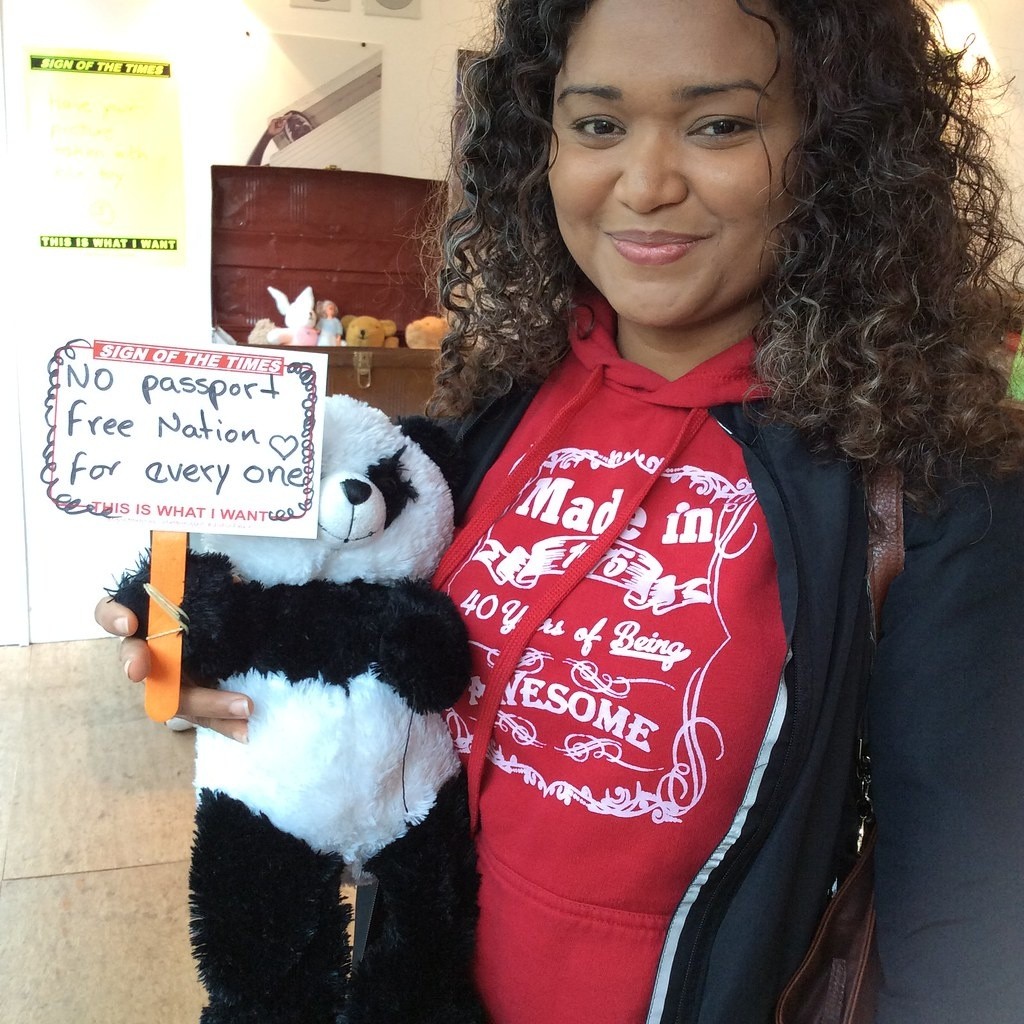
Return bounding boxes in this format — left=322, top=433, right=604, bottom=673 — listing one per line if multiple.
left=96, top=0, right=1024, bottom=1024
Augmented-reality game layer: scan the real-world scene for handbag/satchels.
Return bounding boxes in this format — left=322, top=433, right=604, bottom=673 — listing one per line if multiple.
left=777, top=821, right=879, bottom=1024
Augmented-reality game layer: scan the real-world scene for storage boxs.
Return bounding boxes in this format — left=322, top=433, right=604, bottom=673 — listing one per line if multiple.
left=210, top=164, right=456, bottom=423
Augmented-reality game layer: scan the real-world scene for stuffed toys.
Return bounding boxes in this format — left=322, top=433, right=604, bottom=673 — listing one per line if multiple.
left=249, top=285, right=448, bottom=349
left=115, top=394, right=475, bottom=1024
